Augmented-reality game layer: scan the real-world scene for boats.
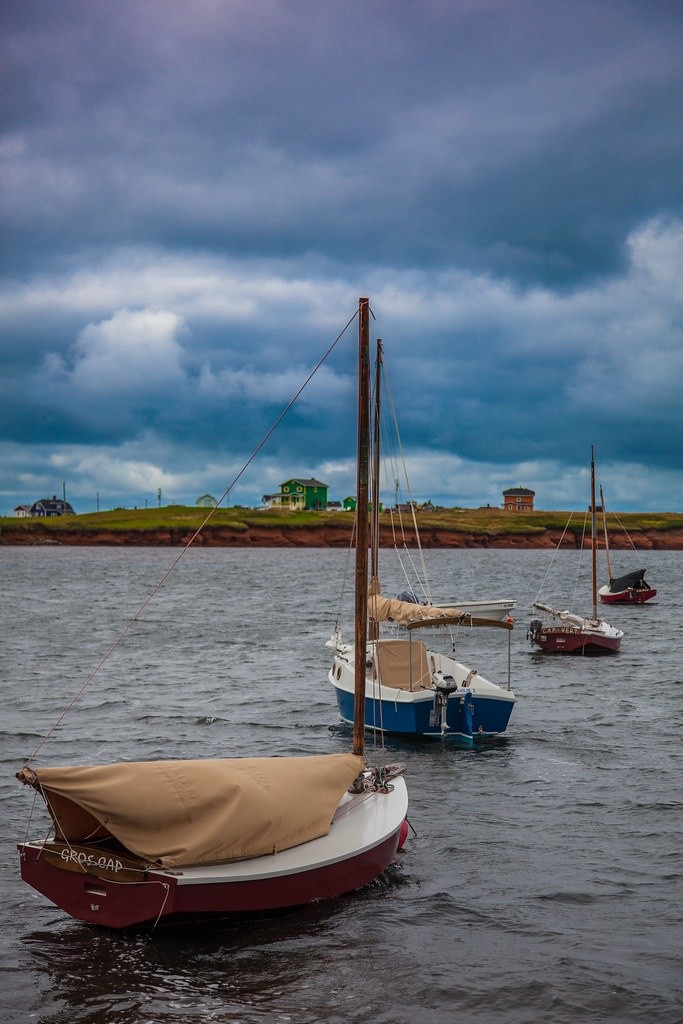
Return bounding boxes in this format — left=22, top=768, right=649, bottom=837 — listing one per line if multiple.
left=397, top=590, right=516, bottom=620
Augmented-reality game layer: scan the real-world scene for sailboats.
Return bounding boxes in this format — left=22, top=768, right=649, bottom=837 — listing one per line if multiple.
left=16, top=299, right=417, bottom=928
left=598, top=484, right=658, bottom=605
left=326, top=339, right=520, bottom=738
left=526, top=443, right=624, bottom=655
